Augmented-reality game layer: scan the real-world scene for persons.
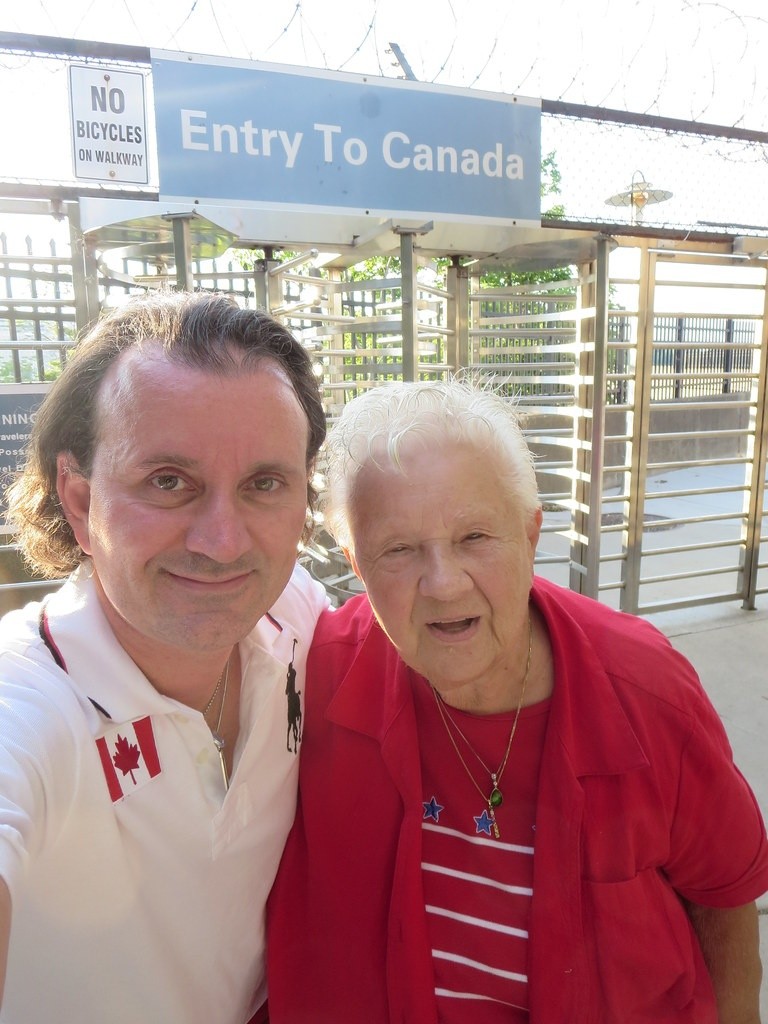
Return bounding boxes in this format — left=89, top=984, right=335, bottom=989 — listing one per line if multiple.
left=0, top=291, right=338, bottom=1024
left=265, top=379, right=768, bottom=1024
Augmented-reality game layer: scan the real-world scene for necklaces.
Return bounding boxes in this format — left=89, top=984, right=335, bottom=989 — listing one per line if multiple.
left=433, top=618, right=532, bottom=838
left=202, top=661, right=230, bottom=791
